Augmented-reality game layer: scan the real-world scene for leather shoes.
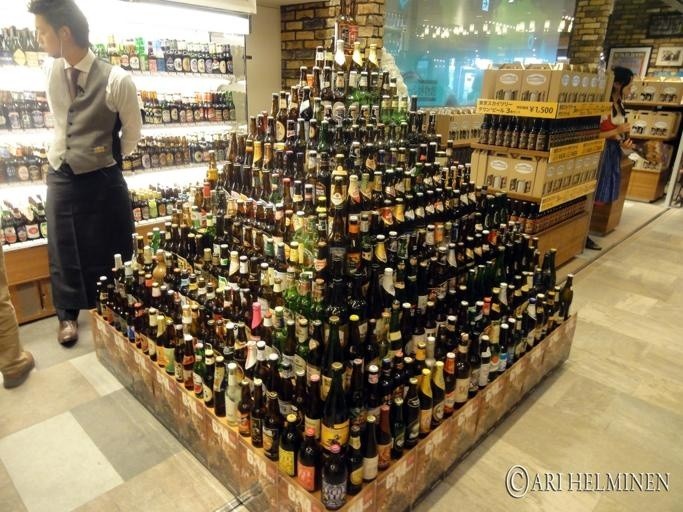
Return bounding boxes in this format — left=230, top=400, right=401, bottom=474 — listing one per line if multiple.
left=58, top=318, right=78, bottom=344
left=4, top=351, right=34, bottom=388
left=586, top=235, right=602, bottom=250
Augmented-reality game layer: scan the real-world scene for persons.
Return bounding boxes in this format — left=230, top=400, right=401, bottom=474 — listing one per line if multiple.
left=26, top=1, right=143, bottom=350
left=0, top=241, right=36, bottom=389
left=584, top=65, right=636, bottom=252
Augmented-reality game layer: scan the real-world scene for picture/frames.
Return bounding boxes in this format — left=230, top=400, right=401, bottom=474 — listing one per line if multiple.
left=606, top=44, right=654, bottom=82
left=654, top=42, right=683, bottom=68
left=645, top=10, right=683, bottom=39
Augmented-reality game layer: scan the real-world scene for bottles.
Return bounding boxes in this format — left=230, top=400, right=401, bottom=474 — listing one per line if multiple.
left=0, top=1, right=601, bottom=511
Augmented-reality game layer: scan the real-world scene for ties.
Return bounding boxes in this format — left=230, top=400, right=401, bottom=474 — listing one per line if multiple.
left=68, top=67, right=79, bottom=102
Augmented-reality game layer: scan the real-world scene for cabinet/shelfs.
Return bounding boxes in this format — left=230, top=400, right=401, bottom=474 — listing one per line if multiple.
left=623, top=81, right=683, bottom=203
left=469, top=71, right=614, bottom=268
left=589, top=158, right=635, bottom=237
left=0, top=63, right=239, bottom=326
